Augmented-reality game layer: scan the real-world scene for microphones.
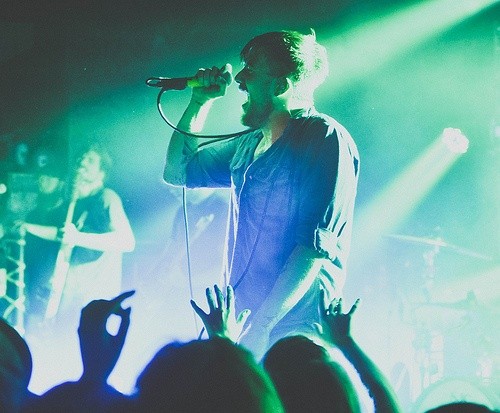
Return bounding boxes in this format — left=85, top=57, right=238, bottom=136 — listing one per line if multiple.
left=158, top=70, right=233, bottom=88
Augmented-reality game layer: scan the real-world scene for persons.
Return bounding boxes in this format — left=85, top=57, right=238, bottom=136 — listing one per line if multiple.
left=163, top=31, right=361, bottom=364
left=16, top=147, right=136, bottom=321
left=1, top=285, right=496, bottom=412
